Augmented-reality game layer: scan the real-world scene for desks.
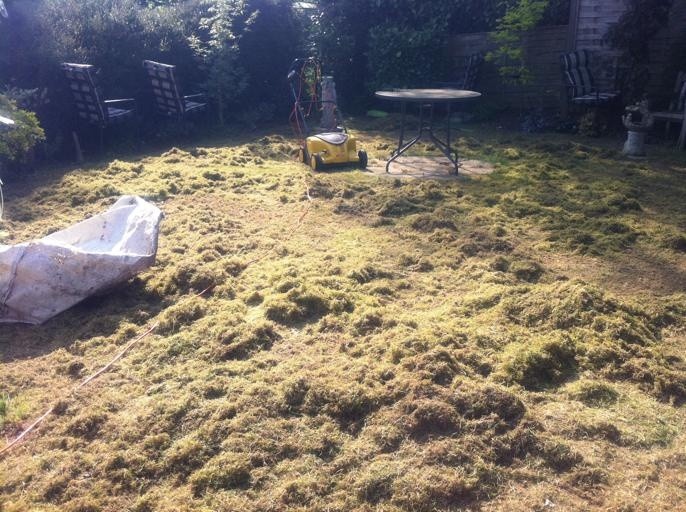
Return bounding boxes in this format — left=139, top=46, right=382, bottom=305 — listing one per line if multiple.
left=375, top=88, right=483, bottom=176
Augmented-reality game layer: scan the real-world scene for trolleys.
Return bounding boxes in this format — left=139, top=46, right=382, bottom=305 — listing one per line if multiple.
left=288, top=54, right=367, bottom=170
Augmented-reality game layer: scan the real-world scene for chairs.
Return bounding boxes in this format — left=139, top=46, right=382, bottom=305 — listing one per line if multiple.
left=143, top=59, right=210, bottom=141
left=652, top=70, right=686, bottom=149
left=560, top=49, right=621, bottom=136
left=428, top=50, right=482, bottom=123
left=60, top=62, right=140, bottom=152
